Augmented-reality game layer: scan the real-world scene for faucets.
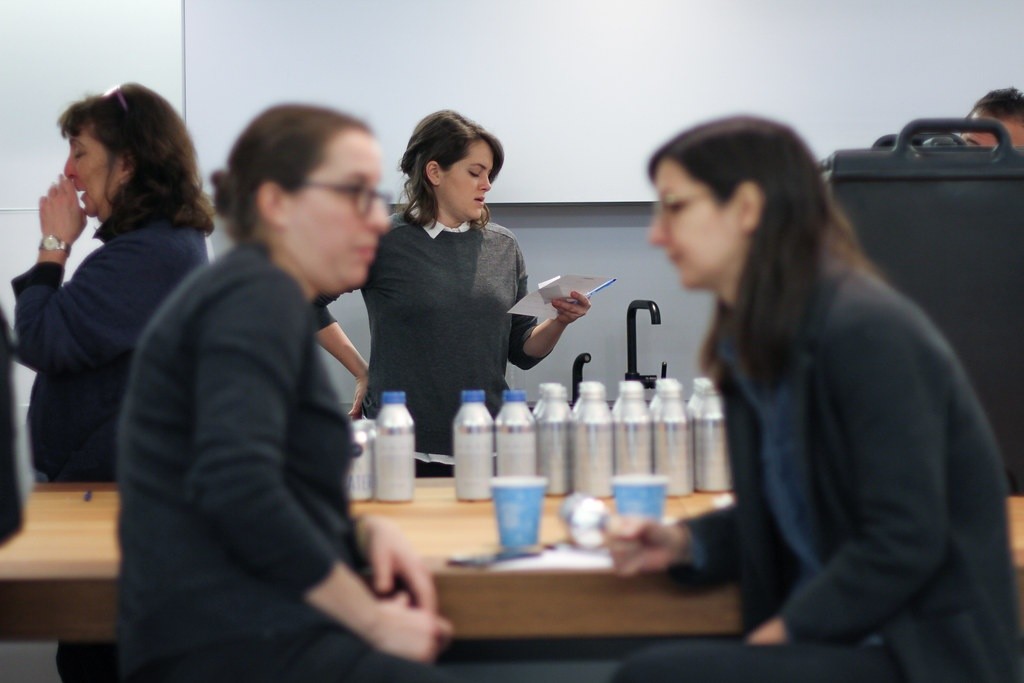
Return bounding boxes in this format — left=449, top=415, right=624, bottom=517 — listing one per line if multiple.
left=624, top=299, right=667, bottom=389
left=572, top=352, right=592, bottom=405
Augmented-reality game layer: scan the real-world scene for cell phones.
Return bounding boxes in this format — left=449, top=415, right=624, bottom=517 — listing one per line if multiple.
left=447, top=550, right=540, bottom=566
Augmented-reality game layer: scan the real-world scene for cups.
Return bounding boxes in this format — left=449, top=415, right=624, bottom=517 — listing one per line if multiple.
left=608, top=474, right=669, bottom=521
left=489, top=475, right=548, bottom=551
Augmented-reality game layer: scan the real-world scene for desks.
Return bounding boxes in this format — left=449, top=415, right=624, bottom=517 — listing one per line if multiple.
left=0, top=483, right=1024, bottom=640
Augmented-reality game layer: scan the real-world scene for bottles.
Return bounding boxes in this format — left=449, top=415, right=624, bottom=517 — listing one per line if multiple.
left=346, top=390, right=418, bottom=503
left=453, top=376, right=734, bottom=496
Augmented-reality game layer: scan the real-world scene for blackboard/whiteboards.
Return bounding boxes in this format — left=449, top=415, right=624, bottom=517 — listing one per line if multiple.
left=178, top=0, right=1024, bottom=214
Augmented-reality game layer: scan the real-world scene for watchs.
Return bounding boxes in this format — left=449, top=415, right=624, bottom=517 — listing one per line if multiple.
left=38, top=234, right=71, bottom=257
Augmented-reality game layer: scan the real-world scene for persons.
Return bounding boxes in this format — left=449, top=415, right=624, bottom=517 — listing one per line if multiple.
left=317, top=109, right=592, bottom=477
left=962, top=88, right=1024, bottom=146
left=606, top=116, right=1024, bottom=683
left=9, top=84, right=213, bottom=682
left=114, top=104, right=453, bottom=683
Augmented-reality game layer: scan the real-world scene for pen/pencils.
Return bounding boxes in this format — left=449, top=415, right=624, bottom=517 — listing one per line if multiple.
left=557, top=279, right=617, bottom=314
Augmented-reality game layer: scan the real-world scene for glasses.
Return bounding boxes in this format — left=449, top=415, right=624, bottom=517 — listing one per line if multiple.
left=104, top=86, right=128, bottom=113
left=288, top=182, right=392, bottom=215
